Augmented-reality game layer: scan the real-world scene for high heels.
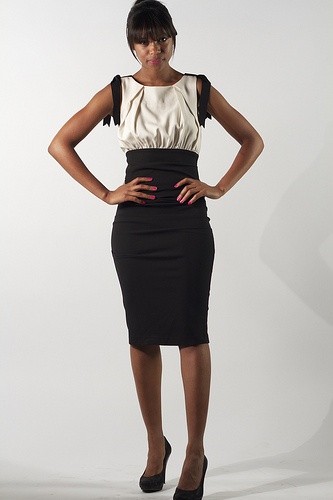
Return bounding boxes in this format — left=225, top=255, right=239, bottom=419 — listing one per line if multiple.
left=172, top=455, right=208, bottom=500
left=139, top=436, right=172, bottom=493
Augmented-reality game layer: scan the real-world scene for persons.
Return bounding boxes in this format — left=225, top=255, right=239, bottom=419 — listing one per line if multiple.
left=47, top=0, right=265, bottom=500
